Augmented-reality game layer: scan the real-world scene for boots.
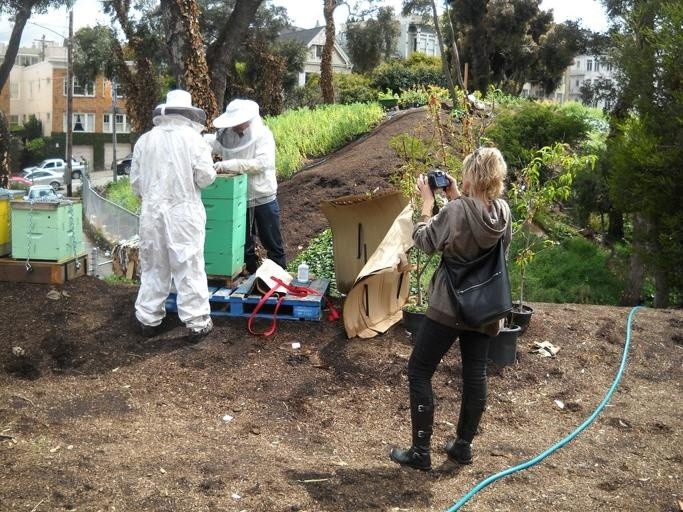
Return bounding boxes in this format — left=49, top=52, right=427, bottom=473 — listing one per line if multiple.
left=444, top=395, right=487, bottom=466
left=387, top=394, right=434, bottom=472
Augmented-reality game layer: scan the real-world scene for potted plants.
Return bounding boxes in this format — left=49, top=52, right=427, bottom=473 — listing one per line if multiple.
left=394, top=164, right=438, bottom=334
left=495, top=195, right=522, bottom=367
left=509, top=142, right=598, bottom=341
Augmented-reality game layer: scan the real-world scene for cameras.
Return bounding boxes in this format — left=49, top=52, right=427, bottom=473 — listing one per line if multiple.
left=427, top=171, right=451, bottom=189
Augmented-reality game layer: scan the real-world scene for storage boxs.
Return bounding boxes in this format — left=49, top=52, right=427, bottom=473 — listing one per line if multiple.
left=0, top=189, right=84, bottom=263
left=200, top=173, right=247, bottom=276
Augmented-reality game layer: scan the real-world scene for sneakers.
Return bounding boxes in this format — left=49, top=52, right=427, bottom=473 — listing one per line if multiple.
left=140, top=317, right=177, bottom=338
left=188, top=316, right=214, bottom=344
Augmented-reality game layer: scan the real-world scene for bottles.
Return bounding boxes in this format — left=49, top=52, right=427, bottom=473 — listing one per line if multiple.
left=298, top=261, right=309, bottom=283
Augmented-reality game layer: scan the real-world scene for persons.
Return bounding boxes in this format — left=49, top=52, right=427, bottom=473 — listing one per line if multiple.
left=200, top=98, right=286, bottom=275
left=129, top=89, right=213, bottom=340
left=388, top=146, right=514, bottom=470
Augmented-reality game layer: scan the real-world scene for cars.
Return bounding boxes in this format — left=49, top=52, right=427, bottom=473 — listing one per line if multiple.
left=9, top=158, right=88, bottom=191
left=111, top=151, right=134, bottom=175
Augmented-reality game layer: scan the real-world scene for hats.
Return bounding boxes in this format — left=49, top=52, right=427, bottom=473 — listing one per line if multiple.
left=151, top=89, right=208, bottom=127
left=211, top=97, right=260, bottom=130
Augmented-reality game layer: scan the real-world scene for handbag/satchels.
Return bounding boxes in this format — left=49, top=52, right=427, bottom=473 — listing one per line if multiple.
left=440, top=231, right=514, bottom=329
